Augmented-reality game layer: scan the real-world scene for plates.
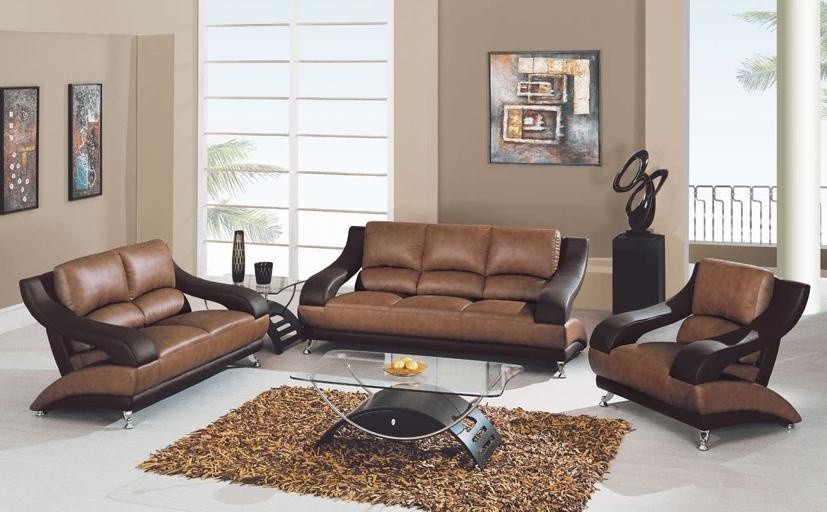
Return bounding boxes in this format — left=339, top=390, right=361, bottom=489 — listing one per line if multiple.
left=382, top=362, right=428, bottom=378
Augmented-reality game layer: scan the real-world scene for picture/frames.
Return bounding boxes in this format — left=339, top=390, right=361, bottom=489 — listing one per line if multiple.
left=488, top=51, right=601, bottom=166
left=0, top=87, right=39, bottom=215
left=68, top=84, right=102, bottom=201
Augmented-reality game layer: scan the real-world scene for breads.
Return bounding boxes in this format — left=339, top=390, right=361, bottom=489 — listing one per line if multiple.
left=393, top=357, right=418, bottom=369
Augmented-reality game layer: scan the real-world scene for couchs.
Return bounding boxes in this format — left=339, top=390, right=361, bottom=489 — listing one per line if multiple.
left=298, top=220, right=590, bottom=378
left=588, top=256, right=810, bottom=451
left=19, top=238, right=270, bottom=429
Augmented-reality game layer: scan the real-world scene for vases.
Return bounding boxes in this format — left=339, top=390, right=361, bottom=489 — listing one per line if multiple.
left=232, top=231, right=245, bottom=282
left=255, top=263, right=273, bottom=283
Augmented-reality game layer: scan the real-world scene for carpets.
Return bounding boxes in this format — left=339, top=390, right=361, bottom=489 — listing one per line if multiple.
left=136, top=385, right=636, bottom=512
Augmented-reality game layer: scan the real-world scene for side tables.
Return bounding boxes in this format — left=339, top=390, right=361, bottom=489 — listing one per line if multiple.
left=204, top=274, right=306, bottom=355
